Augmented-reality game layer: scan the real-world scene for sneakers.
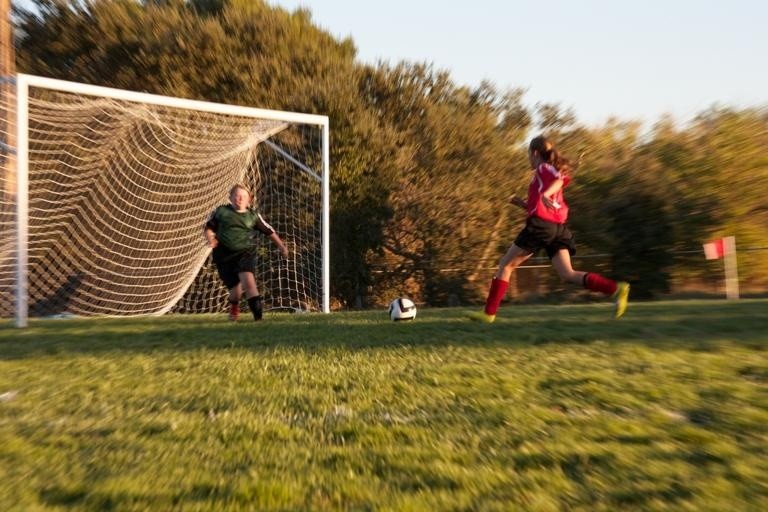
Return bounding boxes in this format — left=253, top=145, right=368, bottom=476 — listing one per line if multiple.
left=467, top=306, right=496, bottom=324
left=231, top=301, right=263, bottom=320
left=612, top=282, right=630, bottom=317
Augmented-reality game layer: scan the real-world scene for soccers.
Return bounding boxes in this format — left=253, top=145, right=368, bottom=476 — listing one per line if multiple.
left=388, top=298, right=417, bottom=321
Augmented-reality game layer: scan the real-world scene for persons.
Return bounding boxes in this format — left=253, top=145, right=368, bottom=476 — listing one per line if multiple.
left=461, top=133, right=631, bottom=326
left=203, top=183, right=289, bottom=321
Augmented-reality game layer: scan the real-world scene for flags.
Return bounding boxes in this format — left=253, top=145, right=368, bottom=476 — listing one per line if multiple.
left=704, top=238, right=732, bottom=262
